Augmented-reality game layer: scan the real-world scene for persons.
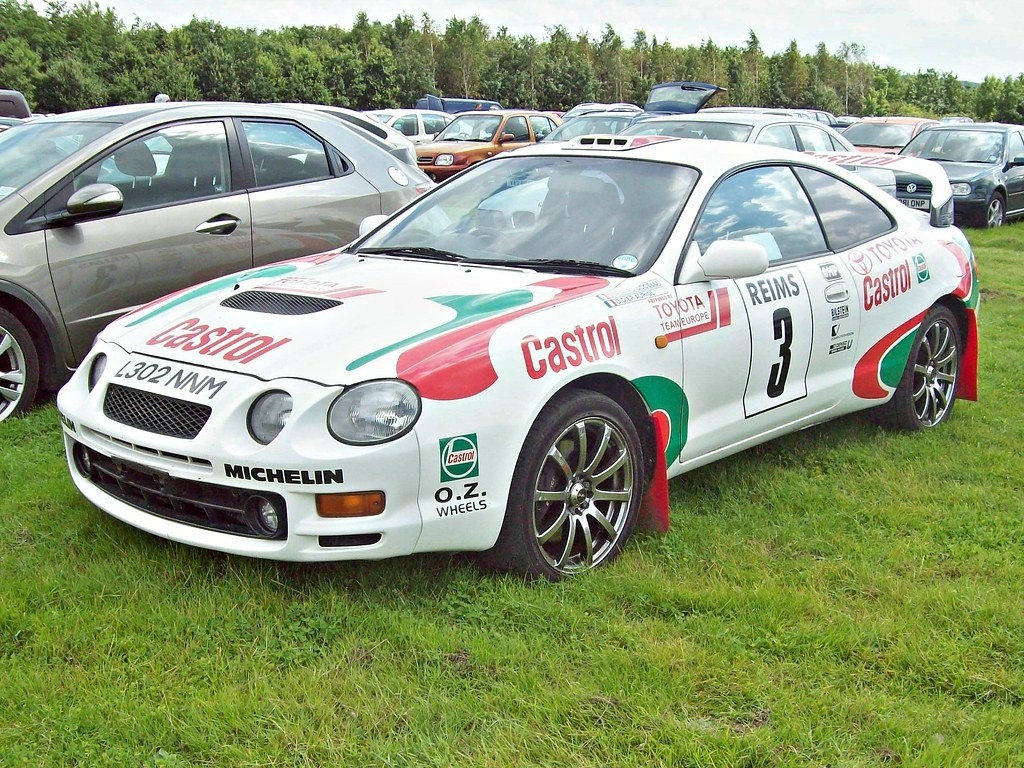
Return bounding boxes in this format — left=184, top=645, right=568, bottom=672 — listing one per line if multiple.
left=155, top=93, right=171, bottom=103
left=474, top=103, right=483, bottom=111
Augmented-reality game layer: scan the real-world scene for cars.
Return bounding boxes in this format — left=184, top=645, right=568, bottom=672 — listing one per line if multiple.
left=0, top=97, right=448, bottom=427
left=265, top=81, right=956, bottom=232
left=890, top=123, right=1024, bottom=228
left=475, top=114, right=864, bottom=235
left=842, top=116, right=941, bottom=162
left=0, top=87, right=311, bottom=186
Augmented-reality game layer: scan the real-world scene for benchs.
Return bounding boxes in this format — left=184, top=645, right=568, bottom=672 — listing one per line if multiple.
left=169, top=142, right=325, bottom=185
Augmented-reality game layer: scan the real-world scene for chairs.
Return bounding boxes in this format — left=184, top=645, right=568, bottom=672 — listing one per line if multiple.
left=402, top=121, right=414, bottom=135
left=592, top=126, right=612, bottom=134
left=562, top=183, right=621, bottom=258
left=485, top=122, right=499, bottom=141
left=169, top=142, right=221, bottom=202
left=114, top=141, right=160, bottom=205
left=942, top=138, right=964, bottom=154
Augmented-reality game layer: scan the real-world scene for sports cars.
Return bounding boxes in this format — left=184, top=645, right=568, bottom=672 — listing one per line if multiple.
left=55, top=132, right=984, bottom=589
